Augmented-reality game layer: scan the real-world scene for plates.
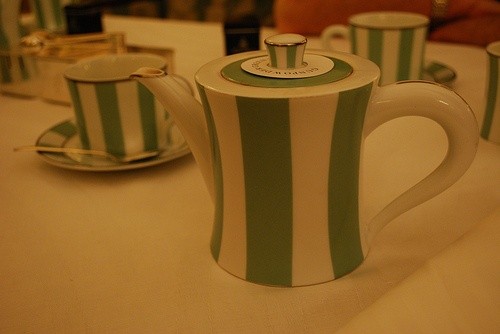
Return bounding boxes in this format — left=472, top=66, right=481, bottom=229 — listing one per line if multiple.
left=35, top=114, right=191, bottom=171
left=425, top=58, right=456, bottom=86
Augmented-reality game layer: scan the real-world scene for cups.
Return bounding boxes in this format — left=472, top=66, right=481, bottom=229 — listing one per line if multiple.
left=322, top=13, right=429, bottom=86
left=0, top=0, right=32, bottom=86
left=30, top=0, right=66, bottom=35
left=223, top=0, right=275, bottom=57
left=66, top=53, right=195, bottom=163
left=482, top=42, right=500, bottom=145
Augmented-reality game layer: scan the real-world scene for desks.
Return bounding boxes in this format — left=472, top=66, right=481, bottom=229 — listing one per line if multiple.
left=0, top=16, right=500, bottom=333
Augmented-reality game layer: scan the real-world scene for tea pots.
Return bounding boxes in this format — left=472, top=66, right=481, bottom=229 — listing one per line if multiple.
left=131, top=32, right=480, bottom=288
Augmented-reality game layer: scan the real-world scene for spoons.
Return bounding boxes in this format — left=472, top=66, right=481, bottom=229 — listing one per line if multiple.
left=14, top=144, right=161, bottom=162
